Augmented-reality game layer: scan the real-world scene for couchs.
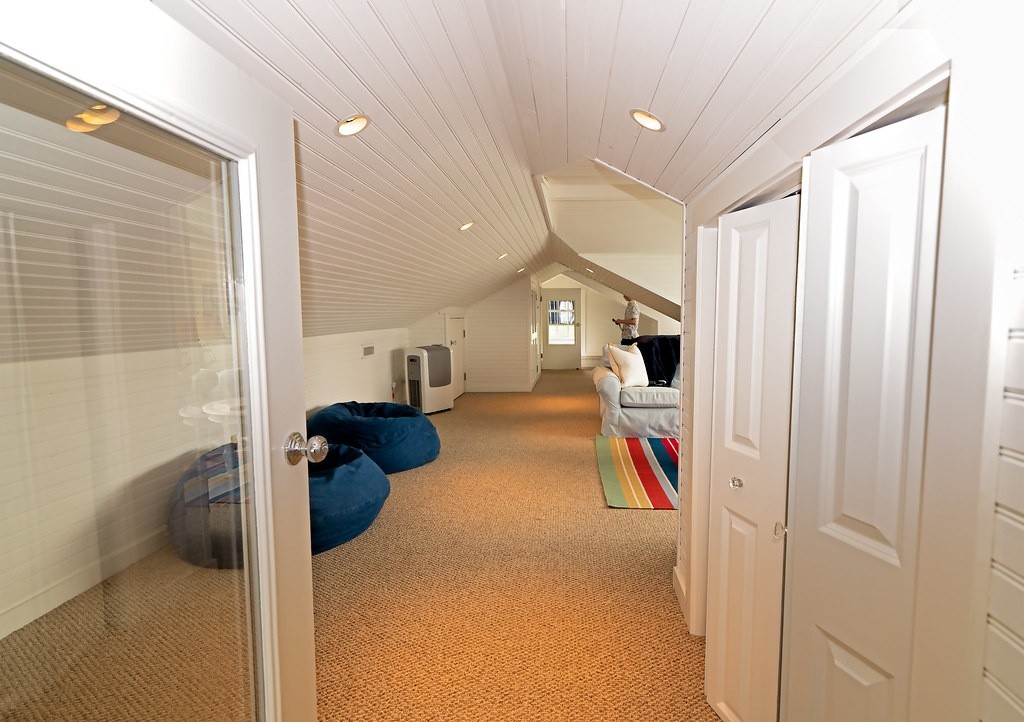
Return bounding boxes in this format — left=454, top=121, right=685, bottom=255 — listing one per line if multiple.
left=590, top=334, right=680, bottom=437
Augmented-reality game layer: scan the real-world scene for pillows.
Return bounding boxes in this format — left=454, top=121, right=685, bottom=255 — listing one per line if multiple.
left=606, top=340, right=652, bottom=387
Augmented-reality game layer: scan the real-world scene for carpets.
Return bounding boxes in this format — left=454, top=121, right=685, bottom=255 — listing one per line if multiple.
left=593, top=432, right=679, bottom=511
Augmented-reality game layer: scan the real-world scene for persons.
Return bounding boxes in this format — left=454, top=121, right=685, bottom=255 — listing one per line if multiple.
left=615, top=294, right=640, bottom=345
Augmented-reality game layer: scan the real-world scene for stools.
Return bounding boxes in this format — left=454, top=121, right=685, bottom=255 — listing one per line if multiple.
left=162, top=440, right=392, bottom=572
left=306, top=398, right=443, bottom=477
